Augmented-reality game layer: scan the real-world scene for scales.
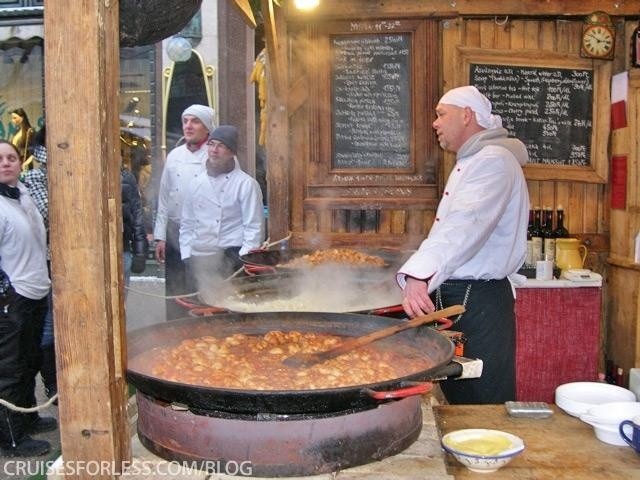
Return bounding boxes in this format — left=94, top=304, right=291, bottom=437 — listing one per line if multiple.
left=564, top=269, right=603, bottom=281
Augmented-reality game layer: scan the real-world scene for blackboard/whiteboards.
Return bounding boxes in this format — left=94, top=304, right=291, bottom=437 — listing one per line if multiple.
left=329, top=31, right=416, bottom=174
left=452, top=47, right=612, bottom=185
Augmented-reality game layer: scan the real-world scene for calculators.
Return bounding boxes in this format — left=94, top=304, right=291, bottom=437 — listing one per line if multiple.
left=505, top=401, right=554, bottom=417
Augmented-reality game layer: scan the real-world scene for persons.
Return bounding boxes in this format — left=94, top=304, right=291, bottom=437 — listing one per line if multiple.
left=394, top=82, right=530, bottom=406
left=179, top=125, right=265, bottom=314
left=0, top=140, right=57, bottom=457
left=11, top=107, right=37, bottom=170
left=119, top=148, right=149, bottom=305
left=25, top=123, right=58, bottom=406
left=154, top=104, right=217, bottom=321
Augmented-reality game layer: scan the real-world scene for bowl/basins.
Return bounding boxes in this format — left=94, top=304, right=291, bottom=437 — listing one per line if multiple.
left=439, top=427, right=526, bottom=475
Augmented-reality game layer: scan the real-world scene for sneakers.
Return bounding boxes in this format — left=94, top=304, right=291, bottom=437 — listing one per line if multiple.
left=28, top=417, right=57, bottom=434
left=2, top=438, right=50, bottom=456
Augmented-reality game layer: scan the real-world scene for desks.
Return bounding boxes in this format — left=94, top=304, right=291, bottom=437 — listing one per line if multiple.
left=431, top=394, right=640, bottom=479
left=510, top=273, right=602, bottom=402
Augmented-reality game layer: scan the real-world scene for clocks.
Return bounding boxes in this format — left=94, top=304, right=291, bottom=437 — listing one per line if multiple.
left=577, top=9, right=618, bottom=61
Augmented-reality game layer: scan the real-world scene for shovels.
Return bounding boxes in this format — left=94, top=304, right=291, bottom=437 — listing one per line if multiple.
left=282, top=304, right=466, bottom=370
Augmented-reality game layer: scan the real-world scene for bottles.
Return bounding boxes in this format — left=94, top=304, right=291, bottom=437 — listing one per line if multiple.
left=555, top=205, right=570, bottom=239
left=524, top=206, right=555, bottom=270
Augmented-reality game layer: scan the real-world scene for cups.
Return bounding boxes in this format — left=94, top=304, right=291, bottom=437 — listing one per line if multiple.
left=535, top=260, right=553, bottom=280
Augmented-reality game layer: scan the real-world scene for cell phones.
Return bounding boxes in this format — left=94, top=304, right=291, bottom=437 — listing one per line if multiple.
left=505, top=399, right=553, bottom=419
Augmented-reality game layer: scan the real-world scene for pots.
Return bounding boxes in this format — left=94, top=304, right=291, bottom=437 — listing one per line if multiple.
left=176, top=272, right=412, bottom=318
left=238, top=245, right=411, bottom=276
left=125, top=312, right=462, bottom=412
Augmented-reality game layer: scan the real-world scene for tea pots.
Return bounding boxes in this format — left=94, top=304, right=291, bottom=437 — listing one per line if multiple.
left=554, top=238, right=588, bottom=278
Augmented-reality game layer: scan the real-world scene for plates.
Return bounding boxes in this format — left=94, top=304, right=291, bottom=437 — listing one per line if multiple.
left=564, top=269, right=602, bottom=283
left=553, top=378, right=640, bottom=456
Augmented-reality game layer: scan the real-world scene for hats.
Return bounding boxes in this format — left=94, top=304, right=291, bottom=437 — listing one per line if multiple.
left=208, top=125, right=238, bottom=152
left=182, top=104, right=215, bottom=132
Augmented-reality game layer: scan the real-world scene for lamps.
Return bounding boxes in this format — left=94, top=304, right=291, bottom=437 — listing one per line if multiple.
left=165, top=35, right=193, bottom=62
